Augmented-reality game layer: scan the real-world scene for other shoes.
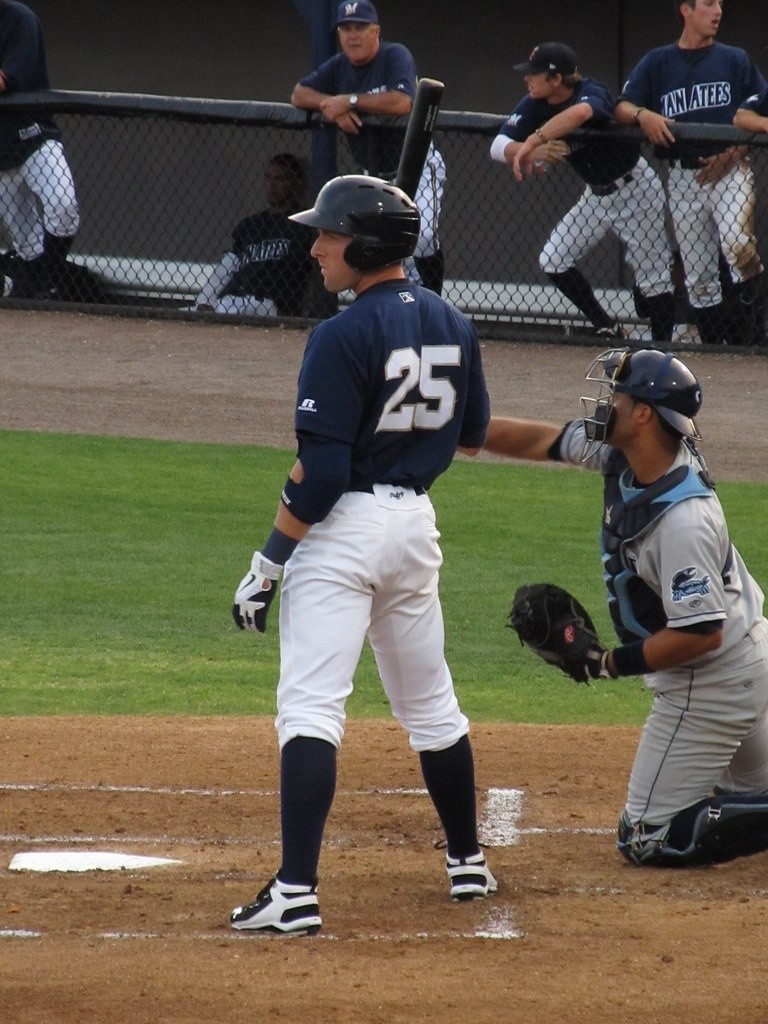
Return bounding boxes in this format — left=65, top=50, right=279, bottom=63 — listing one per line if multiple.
left=48, top=273, right=89, bottom=303
left=5, top=264, right=48, bottom=299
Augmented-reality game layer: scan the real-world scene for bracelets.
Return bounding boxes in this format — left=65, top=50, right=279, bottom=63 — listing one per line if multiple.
left=536, top=126, right=548, bottom=143
left=633, top=106, right=645, bottom=122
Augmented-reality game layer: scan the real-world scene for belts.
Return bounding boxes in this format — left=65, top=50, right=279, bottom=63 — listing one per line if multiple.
left=341, top=481, right=426, bottom=496
left=668, top=158, right=709, bottom=169
left=591, top=174, right=634, bottom=196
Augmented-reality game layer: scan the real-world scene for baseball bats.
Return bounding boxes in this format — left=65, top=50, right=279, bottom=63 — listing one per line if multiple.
left=394, top=76, right=445, bottom=198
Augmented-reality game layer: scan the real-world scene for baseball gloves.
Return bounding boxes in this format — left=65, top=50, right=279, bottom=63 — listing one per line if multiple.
left=499, top=581, right=601, bottom=685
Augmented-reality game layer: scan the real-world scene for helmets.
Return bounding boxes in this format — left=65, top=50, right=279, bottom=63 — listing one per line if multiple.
left=288, top=174, right=422, bottom=274
left=610, top=347, right=703, bottom=441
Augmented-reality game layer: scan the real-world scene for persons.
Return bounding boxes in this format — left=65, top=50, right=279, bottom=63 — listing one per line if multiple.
left=733, top=85, right=768, bottom=132
left=453, top=347, right=768, bottom=866
left=229, top=173, right=497, bottom=933
left=176, top=153, right=338, bottom=318
left=0, top=0, right=99, bottom=300
left=489, top=42, right=676, bottom=343
left=614, top=0, right=768, bottom=352
left=291, top=0, right=446, bottom=298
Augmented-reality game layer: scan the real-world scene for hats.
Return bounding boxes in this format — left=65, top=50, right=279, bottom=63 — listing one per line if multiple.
left=337, top=0, right=378, bottom=24
left=513, top=43, right=578, bottom=75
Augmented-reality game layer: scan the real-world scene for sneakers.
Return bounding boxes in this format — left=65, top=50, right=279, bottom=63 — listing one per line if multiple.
left=230, top=868, right=323, bottom=933
left=435, top=837, right=498, bottom=901
left=591, top=322, right=624, bottom=339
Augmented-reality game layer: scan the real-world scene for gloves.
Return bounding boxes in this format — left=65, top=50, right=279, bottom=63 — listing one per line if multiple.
left=233, top=551, right=284, bottom=633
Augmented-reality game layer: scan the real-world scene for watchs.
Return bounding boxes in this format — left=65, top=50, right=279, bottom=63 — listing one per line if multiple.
left=348, top=92, right=360, bottom=108
